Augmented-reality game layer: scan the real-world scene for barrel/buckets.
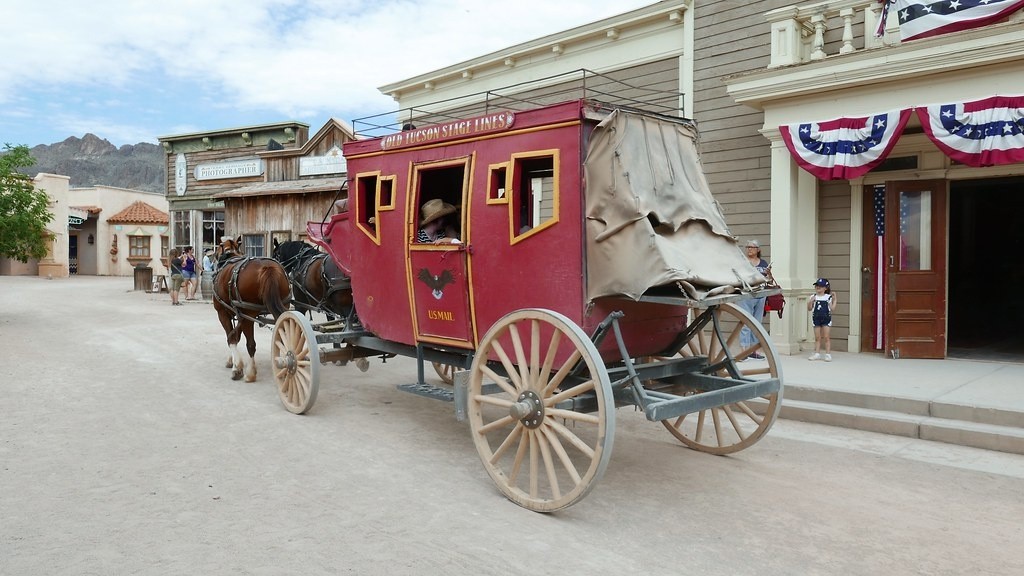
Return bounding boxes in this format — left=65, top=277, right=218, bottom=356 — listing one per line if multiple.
left=201, top=272, right=216, bottom=301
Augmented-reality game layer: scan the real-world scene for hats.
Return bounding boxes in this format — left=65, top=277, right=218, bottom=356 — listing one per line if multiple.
left=813, top=278, right=830, bottom=288
left=419, top=199, right=456, bottom=227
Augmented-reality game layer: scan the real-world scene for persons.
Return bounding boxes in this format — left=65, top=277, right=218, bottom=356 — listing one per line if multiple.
left=366, top=197, right=376, bottom=233
left=202, top=248, right=216, bottom=272
left=807, top=278, right=837, bottom=361
left=416, top=197, right=461, bottom=245
left=737, top=240, right=771, bottom=362
left=180, top=246, right=205, bottom=299
left=169, top=249, right=187, bottom=306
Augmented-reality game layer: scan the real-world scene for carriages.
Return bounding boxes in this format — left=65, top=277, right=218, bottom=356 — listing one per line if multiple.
left=191, top=68, right=786, bottom=514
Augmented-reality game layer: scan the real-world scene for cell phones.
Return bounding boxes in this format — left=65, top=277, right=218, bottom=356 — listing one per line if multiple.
left=767, top=262, right=773, bottom=268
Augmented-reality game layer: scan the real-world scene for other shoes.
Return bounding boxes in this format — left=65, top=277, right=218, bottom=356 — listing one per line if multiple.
left=825, top=354, right=832, bottom=361
left=186, top=296, right=198, bottom=300
left=173, top=301, right=183, bottom=305
left=808, top=353, right=822, bottom=360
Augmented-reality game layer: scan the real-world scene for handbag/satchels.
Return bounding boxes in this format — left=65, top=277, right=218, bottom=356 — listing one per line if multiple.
left=180, top=278, right=186, bottom=287
left=763, top=279, right=786, bottom=319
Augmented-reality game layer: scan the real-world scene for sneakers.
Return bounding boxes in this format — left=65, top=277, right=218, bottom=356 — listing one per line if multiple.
left=739, top=357, right=748, bottom=362
left=748, top=353, right=765, bottom=360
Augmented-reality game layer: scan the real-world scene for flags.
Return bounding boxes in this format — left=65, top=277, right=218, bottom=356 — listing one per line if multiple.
left=897, top=0, right=1024, bottom=42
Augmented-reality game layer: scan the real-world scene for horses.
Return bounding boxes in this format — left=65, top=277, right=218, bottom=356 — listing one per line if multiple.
left=212, top=234, right=369, bottom=383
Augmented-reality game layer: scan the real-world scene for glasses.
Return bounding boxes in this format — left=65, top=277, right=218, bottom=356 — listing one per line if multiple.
left=745, top=246, right=758, bottom=250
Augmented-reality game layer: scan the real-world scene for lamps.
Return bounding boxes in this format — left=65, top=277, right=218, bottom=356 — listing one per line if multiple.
left=87, top=234, right=95, bottom=244
